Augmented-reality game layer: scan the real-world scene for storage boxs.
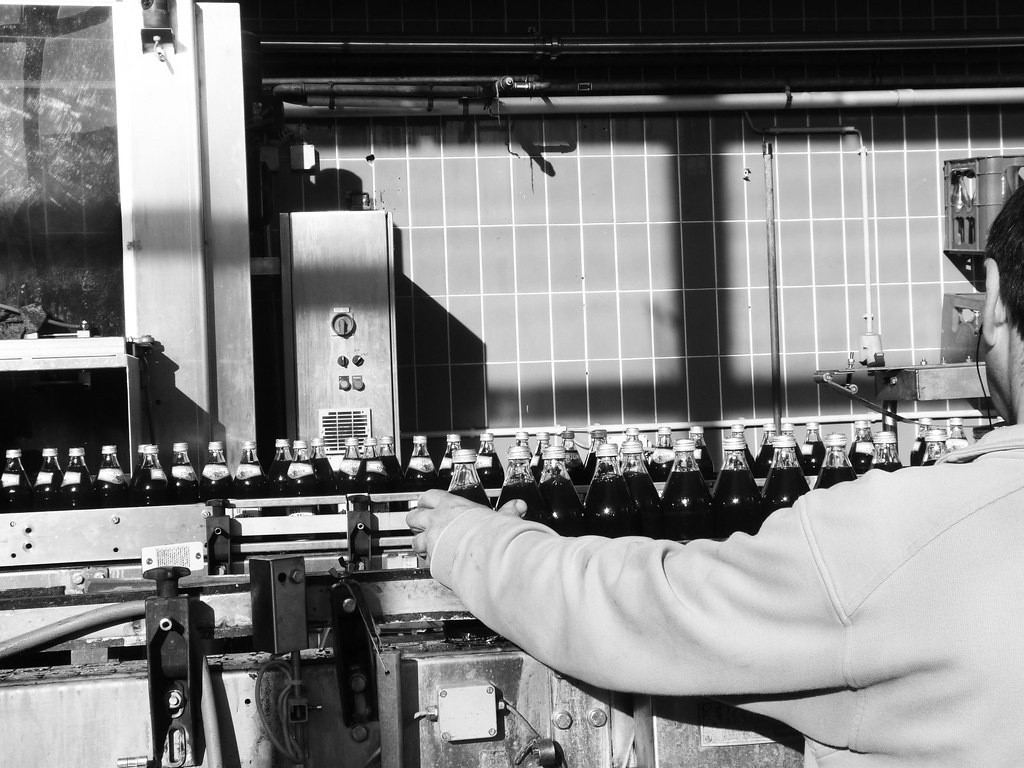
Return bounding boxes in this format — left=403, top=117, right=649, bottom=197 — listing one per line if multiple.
left=942, top=293, right=998, bottom=408
left=941, top=156, right=1023, bottom=256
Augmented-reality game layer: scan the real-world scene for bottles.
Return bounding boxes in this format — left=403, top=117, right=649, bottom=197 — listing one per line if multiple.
left=134, top=416, right=976, bottom=531
left=34, top=444, right=62, bottom=510
left=93, top=446, right=127, bottom=507
left=64, top=449, right=93, bottom=509
left=2, top=448, right=29, bottom=510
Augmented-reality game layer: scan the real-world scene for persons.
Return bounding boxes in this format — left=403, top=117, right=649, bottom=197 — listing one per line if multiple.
left=406, top=179, right=1023, bottom=766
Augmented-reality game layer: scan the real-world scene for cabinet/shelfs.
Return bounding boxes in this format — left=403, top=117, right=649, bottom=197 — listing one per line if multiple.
left=0, top=337, right=154, bottom=476
left=282, top=212, right=402, bottom=462
